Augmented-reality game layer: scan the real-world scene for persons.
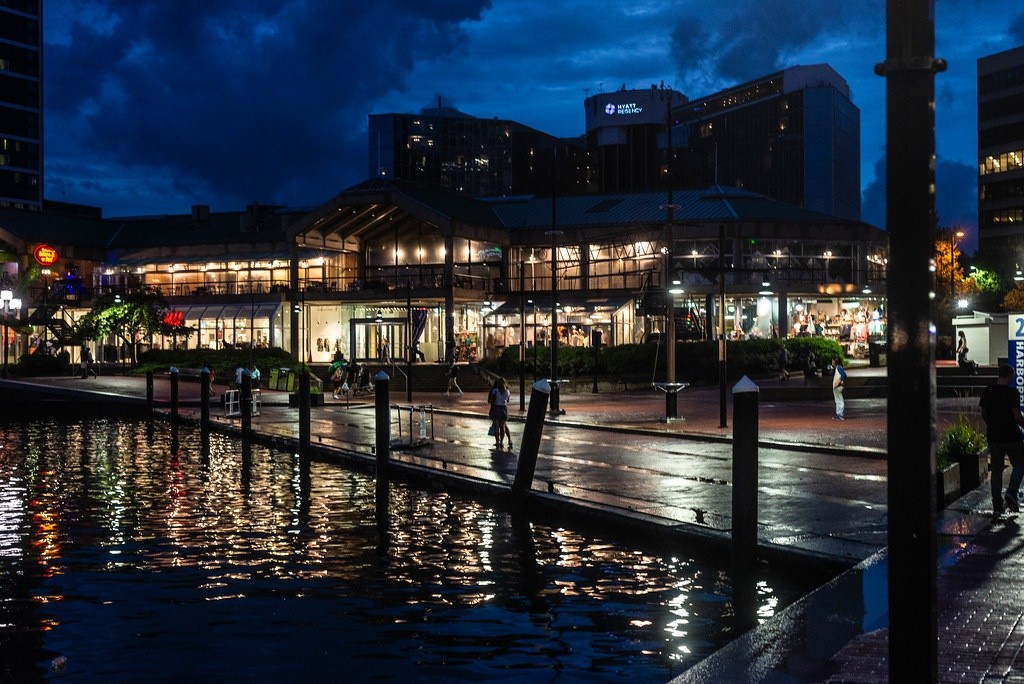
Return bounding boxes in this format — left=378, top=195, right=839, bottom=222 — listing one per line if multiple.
left=80, top=345, right=97, bottom=379
left=203, top=366, right=216, bottom=397
left=443, top=358, right=464, bottom=397
left=832, top=358, right=847, bottom=421
left=488, top=377, right=513, bottom=449
left=332, top=338, right=346, bottom=354
left=778, top=343, right=789, bottom=380
left=957, top=331, right=978, bottom=374
left=805, top=349, right=816, bottom=386
left=331, top=358, right=373, bottom=399
left=979, top=364, right=1024, bottom=513
left=455, top=334, right=471, bottom=352
left=571, top=325, right=585, bottom=348
left=38, top=339, right=44, bottom=355
left=234, top=364, right=260, bottom=395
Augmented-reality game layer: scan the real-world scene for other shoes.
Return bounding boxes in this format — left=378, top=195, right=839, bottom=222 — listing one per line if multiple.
left=831, top=415, right=844, bottom=420
left=333, top=394, right=339, bottom=400
left=459, top=392, right=464, bottom=396
left=993, top=504, right=1002, bottom=511
left=444, top=393, right=449, bottom=396
left=1004, top=491, right=1020, bottom=513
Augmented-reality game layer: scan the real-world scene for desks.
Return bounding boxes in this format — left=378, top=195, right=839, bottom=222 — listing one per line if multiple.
left=217, top=286, right=225, bottom=294
left=167, top=288, right=173, bottom=296
left=244, top=285, right=253, bottom=293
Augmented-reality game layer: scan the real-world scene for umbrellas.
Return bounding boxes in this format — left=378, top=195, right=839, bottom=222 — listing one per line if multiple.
left=327, top=359, right=345, bottom=372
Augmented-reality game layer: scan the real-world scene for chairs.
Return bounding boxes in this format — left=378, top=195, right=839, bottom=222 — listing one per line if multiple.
left=160, top=284, right=262, bottom=295
left=328, top=282, right=337, bottom=292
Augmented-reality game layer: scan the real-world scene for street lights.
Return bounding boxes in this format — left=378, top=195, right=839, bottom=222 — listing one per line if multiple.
left=672, top=223, right=775, bottom=430
left=41, top=268, right=50, bottom=342
left=949, top=232, right=965, bottom=359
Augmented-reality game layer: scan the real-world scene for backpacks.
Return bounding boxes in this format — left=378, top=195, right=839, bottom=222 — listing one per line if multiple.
left=449, top=364, right=460, bottom=378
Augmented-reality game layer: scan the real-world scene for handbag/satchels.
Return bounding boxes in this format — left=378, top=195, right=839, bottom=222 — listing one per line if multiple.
left=342, top=381, right=350, bottom=392
left=251, top=376, right=261, bottom=388
left=212, top=372, right=217, bottom=380
left=488, top=426, right=497, bottom=436
left=490, top=391, right=497, bottom=401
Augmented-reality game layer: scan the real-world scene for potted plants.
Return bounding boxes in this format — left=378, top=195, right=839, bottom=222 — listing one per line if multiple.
left=936, top=442, right=960, bottom=495
left=946, top=416, right=989, bottom=488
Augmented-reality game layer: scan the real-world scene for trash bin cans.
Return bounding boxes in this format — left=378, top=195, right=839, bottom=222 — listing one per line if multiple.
left=268, top=368, right=280, bottom=390
left=869, top=340, right=887, bottom=366
left=277, top=367, right=295, bottom=392
